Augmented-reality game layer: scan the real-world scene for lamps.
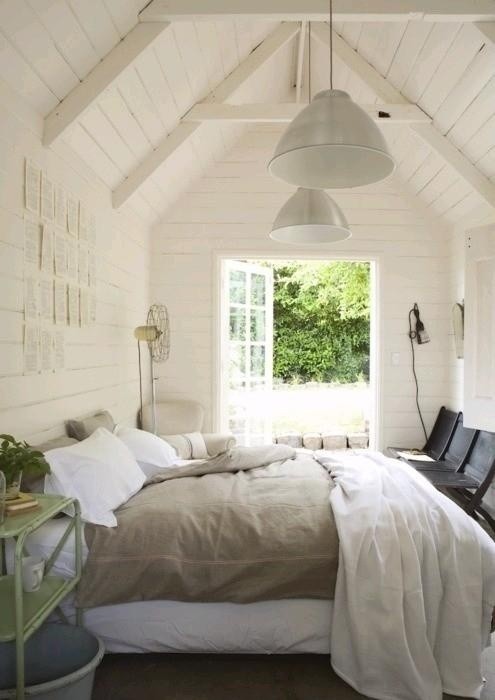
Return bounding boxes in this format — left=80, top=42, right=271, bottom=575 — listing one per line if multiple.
left=267, top=0, right=394, bottom=191
left=267, top=21, right=352, bottom=247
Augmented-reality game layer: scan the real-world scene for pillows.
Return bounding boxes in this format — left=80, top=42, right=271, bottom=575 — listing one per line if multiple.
left=66, top=408, right=115, bottom=439
left=44, top=426, right=147, bottom=527
left=114, top=424, right=175, bottom=477
left=22, top=436, right=80, bottom=491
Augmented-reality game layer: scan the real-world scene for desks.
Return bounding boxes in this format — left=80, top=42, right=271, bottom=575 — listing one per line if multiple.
left=1, top=494, right=84, bottom=700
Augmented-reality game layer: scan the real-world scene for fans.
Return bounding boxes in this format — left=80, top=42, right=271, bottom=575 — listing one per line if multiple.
left=133, top=302, right=171, bottom=434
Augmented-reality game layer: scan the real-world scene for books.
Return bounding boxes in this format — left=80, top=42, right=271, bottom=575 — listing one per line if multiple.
left=2, top=494, right=41, bottom=517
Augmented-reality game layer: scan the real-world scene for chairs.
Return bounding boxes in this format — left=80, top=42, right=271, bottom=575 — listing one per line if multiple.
left=406, top=424, right=479, bottom=473
left=418, top=431, right=495, bottom=533
left=387, top=406, right=462, bottom=463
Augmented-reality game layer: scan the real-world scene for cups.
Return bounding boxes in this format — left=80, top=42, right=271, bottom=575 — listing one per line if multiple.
left=22, top=556, right=45, bottom=594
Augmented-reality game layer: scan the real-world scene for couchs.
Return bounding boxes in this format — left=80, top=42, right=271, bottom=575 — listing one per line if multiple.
left=136, top=401, right=236, bottom=462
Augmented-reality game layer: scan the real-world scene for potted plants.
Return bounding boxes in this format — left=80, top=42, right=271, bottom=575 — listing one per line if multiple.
left=0, top=434, right=52, bottom=500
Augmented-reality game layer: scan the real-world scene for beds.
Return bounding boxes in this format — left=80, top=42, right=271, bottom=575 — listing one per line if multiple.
left=4, top=448, right=494, bottom=657
